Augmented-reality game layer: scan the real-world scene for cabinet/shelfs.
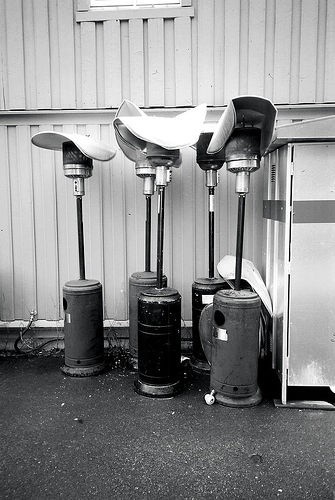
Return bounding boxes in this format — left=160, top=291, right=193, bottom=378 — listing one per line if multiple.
left=262, top=114, right=335, bottom=411
